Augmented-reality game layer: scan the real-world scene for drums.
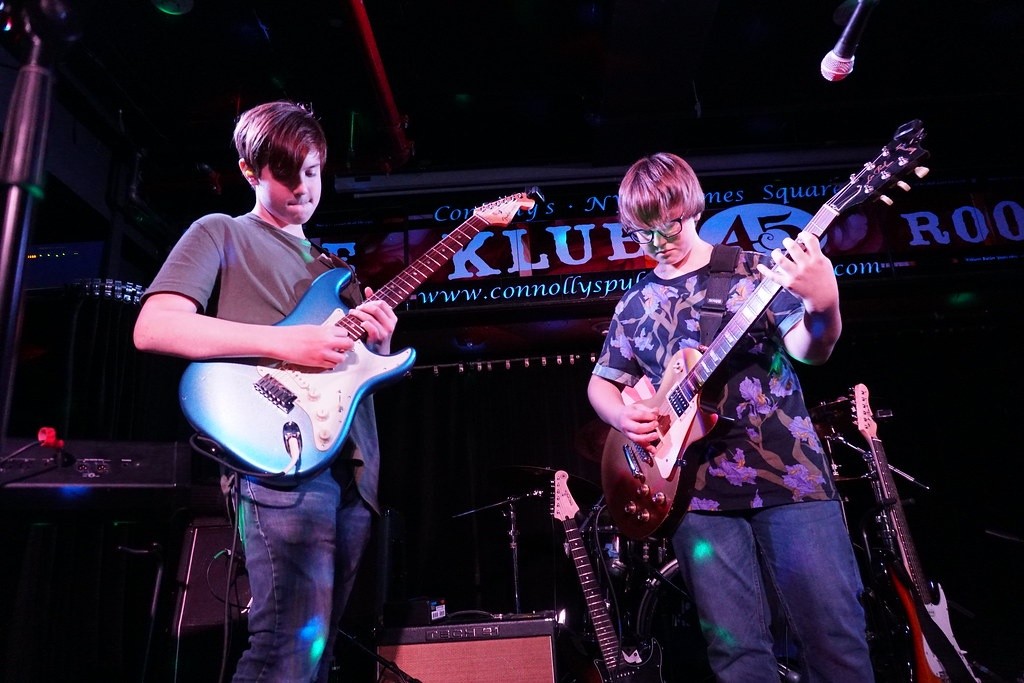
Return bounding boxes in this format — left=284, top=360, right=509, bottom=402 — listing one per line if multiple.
left=575, top=522, right=673, bottom=652
left=635, top=555, right=721, bottom=683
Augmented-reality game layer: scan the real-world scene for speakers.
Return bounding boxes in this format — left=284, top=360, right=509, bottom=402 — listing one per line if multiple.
left=372, top=616, right=586, bottom=683
left=173, top=517, right=258, bottom=642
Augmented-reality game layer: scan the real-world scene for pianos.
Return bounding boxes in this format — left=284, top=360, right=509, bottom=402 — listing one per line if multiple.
left=0, top=433, right=193, bottom=488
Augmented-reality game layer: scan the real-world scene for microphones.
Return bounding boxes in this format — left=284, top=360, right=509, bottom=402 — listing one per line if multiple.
left=821, top=0, right=879, bottom=82
left=223, top=548, right=246, bottom=565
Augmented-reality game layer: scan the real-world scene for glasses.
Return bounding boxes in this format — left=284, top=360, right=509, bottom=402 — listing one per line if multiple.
left=622, top=213, right=686, bottom=245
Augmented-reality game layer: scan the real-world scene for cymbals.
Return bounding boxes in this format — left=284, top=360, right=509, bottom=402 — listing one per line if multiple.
left=833, top=474, right=862, bottom=481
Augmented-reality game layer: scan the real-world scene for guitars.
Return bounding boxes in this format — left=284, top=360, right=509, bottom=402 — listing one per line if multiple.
left=846, top=381, right=980, bottom=683
left=173, top=187, right=540, bottom=495
left=547, top=467, right=669, bottom=683
left=597, top=114, right=938, bottom=546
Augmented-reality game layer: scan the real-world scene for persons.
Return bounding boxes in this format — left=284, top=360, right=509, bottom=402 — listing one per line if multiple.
left=587, top=153, right=875, bottom=683
left=131, top=102, right=397, bottom=683
